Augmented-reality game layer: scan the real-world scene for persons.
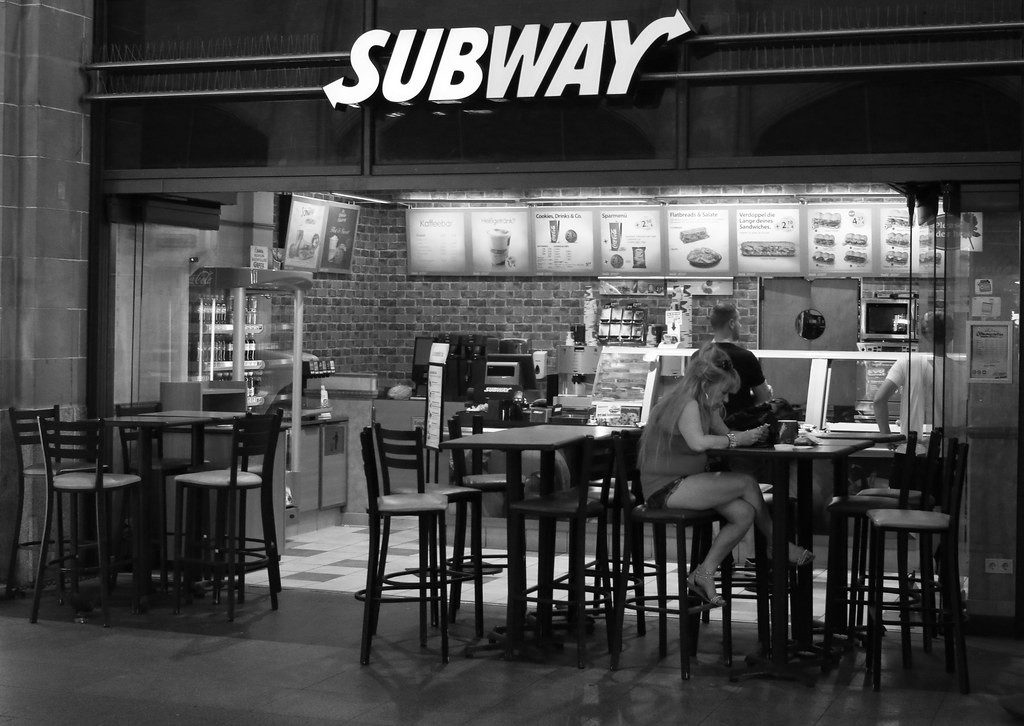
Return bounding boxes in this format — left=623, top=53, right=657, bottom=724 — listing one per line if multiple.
left=639, top=347, right=814, bottom=608
left=873, top=312, right=968, bottom=602
left=690, top=306, right=774, bottom=569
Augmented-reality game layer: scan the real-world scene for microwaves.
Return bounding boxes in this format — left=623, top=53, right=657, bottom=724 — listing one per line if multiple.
left=859, top=297, right=920, bottom=342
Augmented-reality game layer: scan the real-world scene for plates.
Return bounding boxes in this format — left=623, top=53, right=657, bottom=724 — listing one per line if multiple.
left=774, top=444, right=794, bottom=450
left=794, top=445, right=813, bottom=449
left=686, top=254, right=722, bottom=268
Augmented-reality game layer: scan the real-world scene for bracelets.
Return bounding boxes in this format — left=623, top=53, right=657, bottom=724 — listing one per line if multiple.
left=727, top=434, right=737, bottom=448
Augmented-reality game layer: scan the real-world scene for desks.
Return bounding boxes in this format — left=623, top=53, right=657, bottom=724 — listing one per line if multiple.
left=83, top=415, right=210, bottom=613
left=438, top=424, right=642, bottom=660
left=142, top=410, right=250, bottom=598
left=731, top=433, right=878, bottom=683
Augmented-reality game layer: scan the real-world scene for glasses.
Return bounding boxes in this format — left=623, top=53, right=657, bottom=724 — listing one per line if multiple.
left=717, top=360, right=731, bottom=371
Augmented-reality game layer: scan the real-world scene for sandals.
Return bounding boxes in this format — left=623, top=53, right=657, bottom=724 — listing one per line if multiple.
left=687, top=564, right=726, bottom=606
left=767, top=541, right=815, bottom=566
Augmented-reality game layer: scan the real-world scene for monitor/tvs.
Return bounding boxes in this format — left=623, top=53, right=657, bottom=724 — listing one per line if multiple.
left=411, top=335, right=438, bottom=368
left=859, top=297, right=914, bottom=341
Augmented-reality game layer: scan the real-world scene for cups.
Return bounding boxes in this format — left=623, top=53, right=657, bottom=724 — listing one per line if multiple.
left=549, top=220, right=562, bottom=243
left=609, top=221, right=623, bottom=251
left=327, top=237, right=339, bottom=262
left=485, top=227, right=512, bottom=266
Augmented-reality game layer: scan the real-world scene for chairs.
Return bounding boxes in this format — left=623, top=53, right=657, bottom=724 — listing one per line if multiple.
left=610, top=427, right=733, bottom=680
left=553, top=428, right=649, bottom=637
left=511, top=428, right=623, bottom=657
left=115, top=402, right=220, bottom=594
left=852, top=429, right=940, bottom=632
left=826, top=427, right=944, bottom=645
left=446, top=415, right=528, bottom=622
left=5, top=405, right=99, bottom=601
left=358, top=425, right=452, bottom=665
left=30, top=416, right=150, bottom=628
left=376, top=424, right=485, bottom=642
left=173, top=407, right=286, bottom=624
left=866, top=436, right=970, bottom=692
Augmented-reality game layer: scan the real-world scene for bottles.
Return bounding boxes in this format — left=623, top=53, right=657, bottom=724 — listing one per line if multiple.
left=191, top=333, right=256, bottom=362
left=318, top=385, right=331, bottom=419
left=188, top=371, right=254, bottom=396
left=190, top=292, right=226, bottom=324
